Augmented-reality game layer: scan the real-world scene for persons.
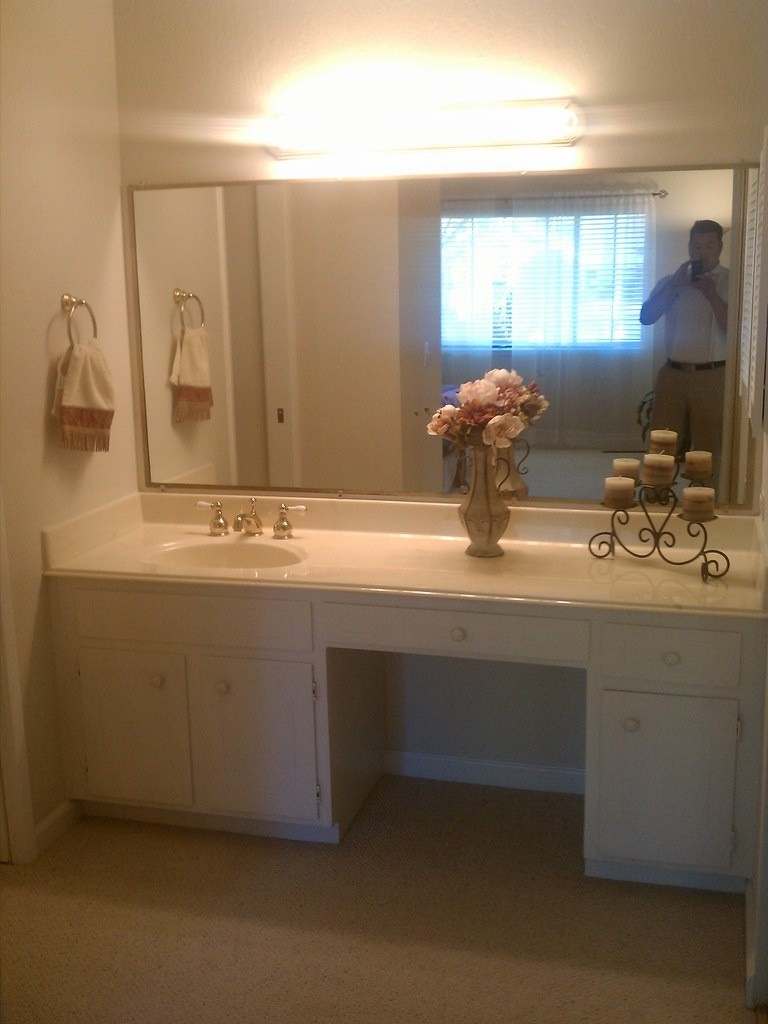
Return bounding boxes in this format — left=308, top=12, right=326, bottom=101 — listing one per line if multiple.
left=640, top=220, right=729, bottom=503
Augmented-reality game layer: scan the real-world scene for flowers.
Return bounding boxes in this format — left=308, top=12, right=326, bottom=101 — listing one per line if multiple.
left=426, top=380, right=523, bottom=449
left=466, top=367, right=551, bottom=432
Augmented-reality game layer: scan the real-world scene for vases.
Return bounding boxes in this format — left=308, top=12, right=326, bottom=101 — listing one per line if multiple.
left=456, top=445, right=513, bottom=560
left=493, top=436, right=536, bottom=498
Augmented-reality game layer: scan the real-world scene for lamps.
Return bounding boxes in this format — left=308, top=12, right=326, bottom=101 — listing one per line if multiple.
left=267, top=95, right=593, bottom=160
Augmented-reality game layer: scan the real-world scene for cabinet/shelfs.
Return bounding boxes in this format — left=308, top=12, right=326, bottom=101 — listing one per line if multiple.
left=45, top=575, right=768, bottom=899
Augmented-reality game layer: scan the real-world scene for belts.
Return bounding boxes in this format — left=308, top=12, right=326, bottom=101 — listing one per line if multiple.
left=666, top=359, right=726, bottom=371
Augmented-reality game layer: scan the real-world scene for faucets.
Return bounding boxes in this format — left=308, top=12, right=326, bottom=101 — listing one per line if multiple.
left=233, top=498, right=264, bottom=538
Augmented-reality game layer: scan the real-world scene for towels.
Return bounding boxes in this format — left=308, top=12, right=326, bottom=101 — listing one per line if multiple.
left=168, top=326, right=216, bottom=422
left=50, top=343, right=116, bottom=453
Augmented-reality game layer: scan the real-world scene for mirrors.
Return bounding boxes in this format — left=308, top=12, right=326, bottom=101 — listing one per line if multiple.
left=126, top=164, right=738, bottom=502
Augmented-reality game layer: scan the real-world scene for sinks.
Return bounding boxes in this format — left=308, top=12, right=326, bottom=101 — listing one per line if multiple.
left=153, top=537, right=309, bottom=571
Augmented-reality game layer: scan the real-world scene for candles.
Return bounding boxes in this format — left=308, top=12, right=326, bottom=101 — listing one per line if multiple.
left=602, top=475, right=636, bottom=509
left=649, top=424, right=679, bottom=455
left=642, top=450, right=677, bottom=486
left=684, top=449, right=714, bottom=479
left=611, top=456, right=640, bottom=487
left=681, top=484, right=718, bottom=521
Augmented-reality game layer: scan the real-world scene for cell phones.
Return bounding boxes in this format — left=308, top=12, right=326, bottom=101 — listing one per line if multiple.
left=691, top=260, right=703, bottom=282
